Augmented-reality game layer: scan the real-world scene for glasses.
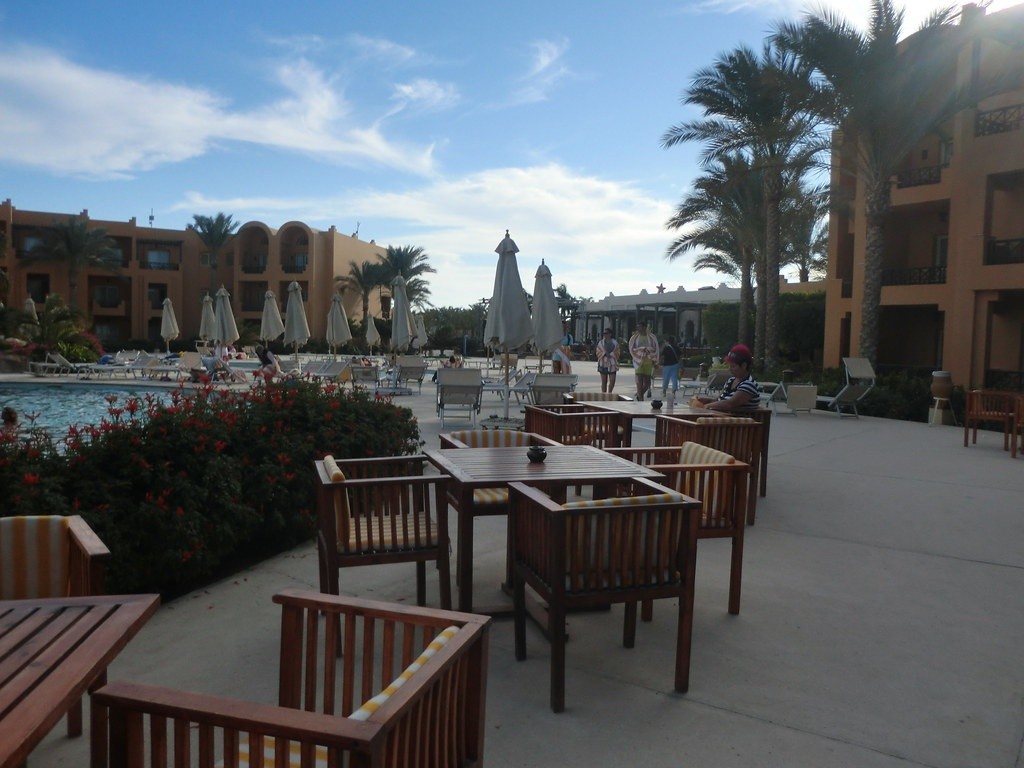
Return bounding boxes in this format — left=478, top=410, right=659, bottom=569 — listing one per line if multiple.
left=602, top=332, right=607, bottom=334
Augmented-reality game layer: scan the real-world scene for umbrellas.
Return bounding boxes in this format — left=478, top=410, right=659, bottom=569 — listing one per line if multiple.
left=365, top=313, right=381, bottom=361
left=24, top=292, right=38, bottom=321
left=214, top=283, right=240, bottom=359
left=160, top=297, right=179, bottom=377
left=199, top=292, right=215, bottom=346
left=326, top=291, right=353, bottom=360
left=390, top=269, right=416, bottom=388
left=412, top=314, right=428, bottom=354
left=260, top=288, right=285, bottom=349
left=483, top=229, right=565, bottom=420
left=283, top=277, right=310, bottom=364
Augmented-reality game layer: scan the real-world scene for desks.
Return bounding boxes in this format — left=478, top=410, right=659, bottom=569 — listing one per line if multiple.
left=421, top=444, right=667, bottom=612
left=574, top=399, right=728, bottom=447
left=0, top=593, right=162, bottom=768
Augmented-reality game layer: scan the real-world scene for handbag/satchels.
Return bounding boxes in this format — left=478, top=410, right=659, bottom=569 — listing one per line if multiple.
left=634, top=356, right=653, bottom=376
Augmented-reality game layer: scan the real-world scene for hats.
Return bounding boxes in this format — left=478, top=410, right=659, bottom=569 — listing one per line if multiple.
left=724, top=344, right=752, bottom=361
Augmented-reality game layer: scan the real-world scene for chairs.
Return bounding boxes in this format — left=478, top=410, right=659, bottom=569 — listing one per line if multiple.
left=0, top=334, right=1024, bottom=768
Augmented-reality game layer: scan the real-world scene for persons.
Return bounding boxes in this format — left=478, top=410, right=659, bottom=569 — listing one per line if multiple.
left=552, top=321, right=573, bottom=374
left=597, top=327, right=620, bottom=393
left=697, top=344, right=760, bottom=419
left=629, top=322, right=659, bottom=401
left=255, top=346, right=280, bottom=385
left=661, top=336, right=681, bottom=398
